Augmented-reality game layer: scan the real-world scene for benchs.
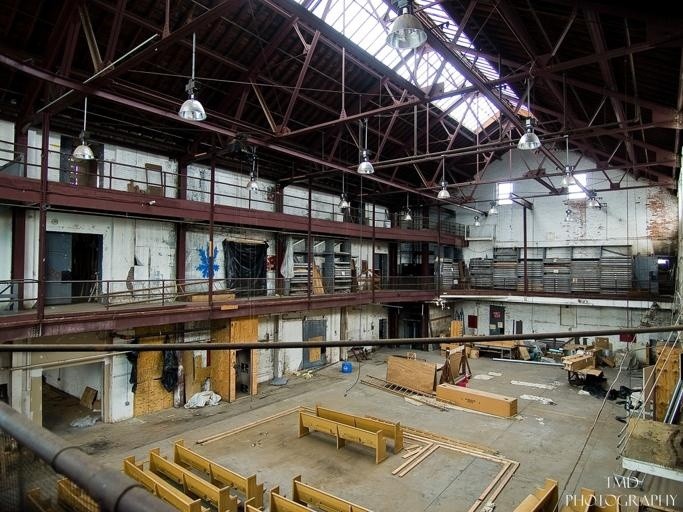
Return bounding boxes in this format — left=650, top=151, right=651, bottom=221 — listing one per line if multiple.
left=297, top=403, right=404, bottom=464
left=511, top=478, right=624, bottom=511
left=16, top=439, right=375, bottom=512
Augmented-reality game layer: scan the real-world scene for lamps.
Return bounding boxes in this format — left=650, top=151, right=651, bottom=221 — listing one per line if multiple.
left=67, top=4, right=601, bottom=228
